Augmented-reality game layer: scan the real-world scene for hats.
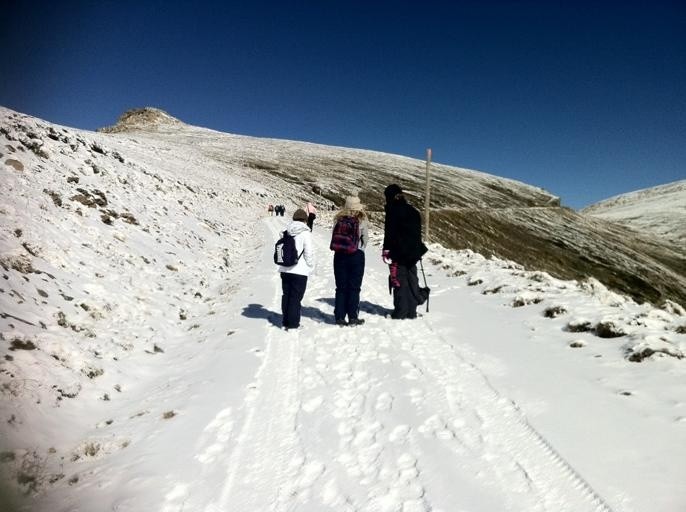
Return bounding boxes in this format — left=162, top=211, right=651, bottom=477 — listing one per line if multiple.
left=294, top=209, right=308, bottom=222
left=345, top=187, right=361, bottom=211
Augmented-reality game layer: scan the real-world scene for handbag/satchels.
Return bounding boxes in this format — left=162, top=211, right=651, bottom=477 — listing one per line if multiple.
left=418, top=259, right=430, bottom=312
left=309, top=213, right=316, bottom=220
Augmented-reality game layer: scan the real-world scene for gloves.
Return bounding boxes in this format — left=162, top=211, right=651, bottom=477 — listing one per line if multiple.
left=382, top=250, right=393, bottom=265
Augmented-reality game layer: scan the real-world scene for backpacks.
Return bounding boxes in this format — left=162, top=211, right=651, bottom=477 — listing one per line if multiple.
left=274, top=229, right=304, bottom=267
left=329, top=215, right=363, bottom=256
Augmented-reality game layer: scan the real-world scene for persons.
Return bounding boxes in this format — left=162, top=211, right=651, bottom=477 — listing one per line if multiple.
left=334, top=195, right=369, bottom=325
left=279, top=209, right=316, bottom=332
left=382, top=184, right=428, bottom=319
left=268, top=204, right=285, bottom=216
left=306, top=202, right=316, bottom=232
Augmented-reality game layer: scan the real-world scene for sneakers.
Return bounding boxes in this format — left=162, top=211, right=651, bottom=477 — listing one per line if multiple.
left=385, top=311, right=407, bottom=319
left=348, top=318, right=364, bottom=327
left=335, top=319, right=348, bottom=327
left=408, top=310, right=417, bottom=319
